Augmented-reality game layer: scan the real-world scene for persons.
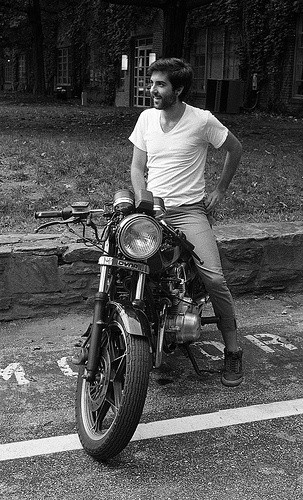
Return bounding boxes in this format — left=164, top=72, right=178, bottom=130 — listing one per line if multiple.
left=128, top=58, right=244, bottom=387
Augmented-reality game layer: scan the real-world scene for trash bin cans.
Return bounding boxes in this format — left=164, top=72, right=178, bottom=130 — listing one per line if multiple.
left=204, top=78, right=242, bottom=114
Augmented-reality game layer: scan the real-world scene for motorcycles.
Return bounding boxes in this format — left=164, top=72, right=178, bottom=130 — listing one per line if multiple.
left=32, top=188, right=223, bottom=462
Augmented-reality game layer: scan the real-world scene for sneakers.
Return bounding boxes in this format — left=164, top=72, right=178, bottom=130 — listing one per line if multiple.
left=222, top=346, right=244, bottom=386
